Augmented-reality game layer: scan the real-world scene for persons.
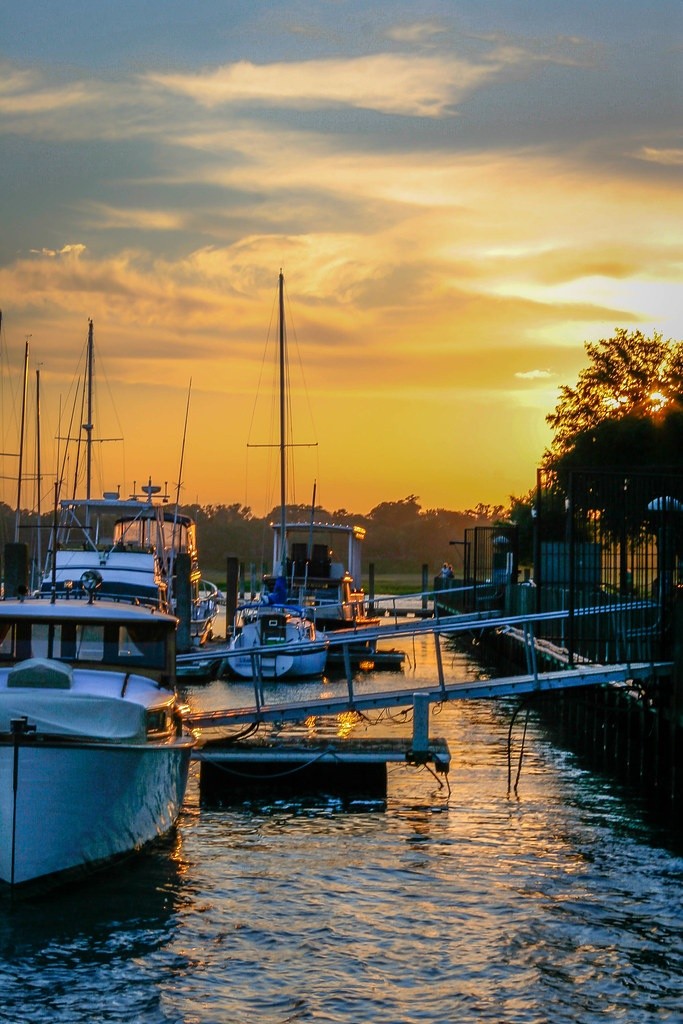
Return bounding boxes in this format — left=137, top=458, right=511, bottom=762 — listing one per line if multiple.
left=438, top=562, right=454, bottom=579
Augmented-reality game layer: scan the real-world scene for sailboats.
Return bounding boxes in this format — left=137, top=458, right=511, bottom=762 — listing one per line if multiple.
left=212, top=266, right=408, bottom=676
left=29, top=320, right=227, bottom=683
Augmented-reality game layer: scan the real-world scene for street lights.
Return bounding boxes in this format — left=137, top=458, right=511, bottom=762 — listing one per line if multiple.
left=449, top=541, right=470, bottom=587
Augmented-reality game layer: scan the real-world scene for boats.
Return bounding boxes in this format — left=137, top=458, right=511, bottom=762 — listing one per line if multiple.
left=0, top=596, right=198, bottom=897
